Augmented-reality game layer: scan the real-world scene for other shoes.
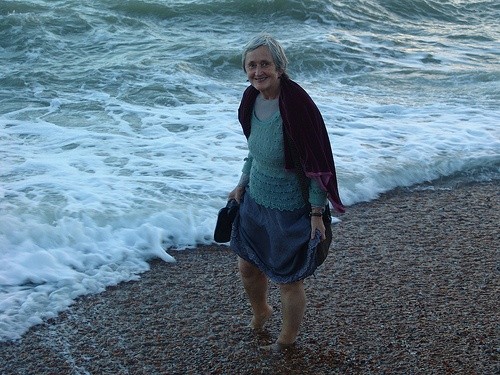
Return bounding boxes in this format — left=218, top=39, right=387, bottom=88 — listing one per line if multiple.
left=213, top=198, right=239, bottom=243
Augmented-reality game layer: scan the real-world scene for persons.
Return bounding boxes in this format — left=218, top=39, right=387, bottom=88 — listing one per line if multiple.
left=212, top=33, right=347, bottom=355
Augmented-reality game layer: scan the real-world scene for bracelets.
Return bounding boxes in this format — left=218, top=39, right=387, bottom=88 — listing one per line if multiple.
left=309, top=212, right=323, bottom=217
